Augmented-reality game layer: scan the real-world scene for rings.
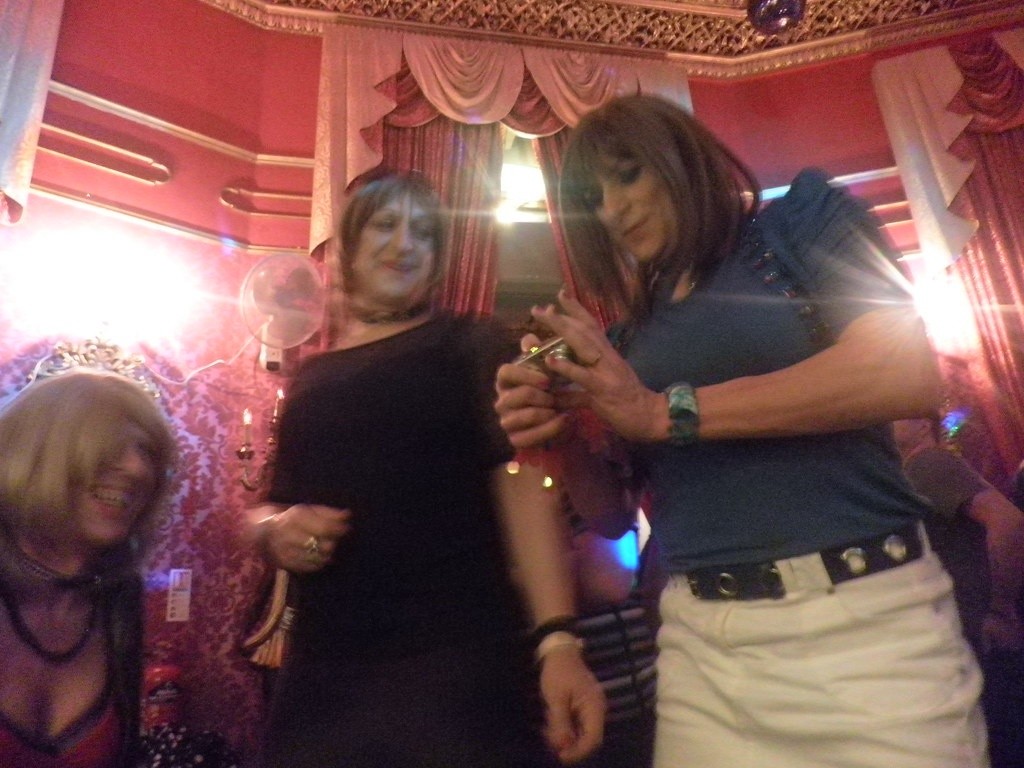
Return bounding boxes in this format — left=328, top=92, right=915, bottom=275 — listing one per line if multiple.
left=304, top=536, right=318, bottom=549
left=304, top=550, right=320, bottom=566
left=590, top=352, right=602, bottom=367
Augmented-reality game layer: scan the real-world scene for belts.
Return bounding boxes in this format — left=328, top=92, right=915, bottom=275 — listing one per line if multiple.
left=686, top=524, right=925, bottom=599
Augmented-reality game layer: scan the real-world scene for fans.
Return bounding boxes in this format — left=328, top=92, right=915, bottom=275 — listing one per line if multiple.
left=241, top=253, right=324, bottom=369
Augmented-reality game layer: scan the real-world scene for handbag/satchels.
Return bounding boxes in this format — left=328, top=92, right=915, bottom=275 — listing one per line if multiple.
left=239, top=561, right=296, bottom=669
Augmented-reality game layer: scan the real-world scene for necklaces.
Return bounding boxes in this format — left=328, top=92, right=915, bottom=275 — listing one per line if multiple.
left=350, top=303, right=432, bottom=325
left=0, top=580, right=100, bottom=665
left=8, top=540, right=74, bottom=585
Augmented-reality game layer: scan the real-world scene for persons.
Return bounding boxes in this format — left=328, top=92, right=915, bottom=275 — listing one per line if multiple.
left=890, top=407, right=1024, bottom=676
left=491, top=92, right=994, bottom=768
left=0, top=368, right=178, bottom=768
left=244, top=171, right=606, bottom=768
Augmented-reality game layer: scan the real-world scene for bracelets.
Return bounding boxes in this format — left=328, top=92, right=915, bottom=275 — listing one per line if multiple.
left=665, top=381, right=700, bottom=447
left=532, top=616, right=588, bottom=663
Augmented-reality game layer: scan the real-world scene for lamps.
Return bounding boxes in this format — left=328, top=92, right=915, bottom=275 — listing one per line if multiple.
left=237, top=389, right=285, bottom=491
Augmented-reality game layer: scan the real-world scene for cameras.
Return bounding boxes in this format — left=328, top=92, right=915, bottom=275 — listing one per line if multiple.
left=512, top=335, right=579, bottom=392
